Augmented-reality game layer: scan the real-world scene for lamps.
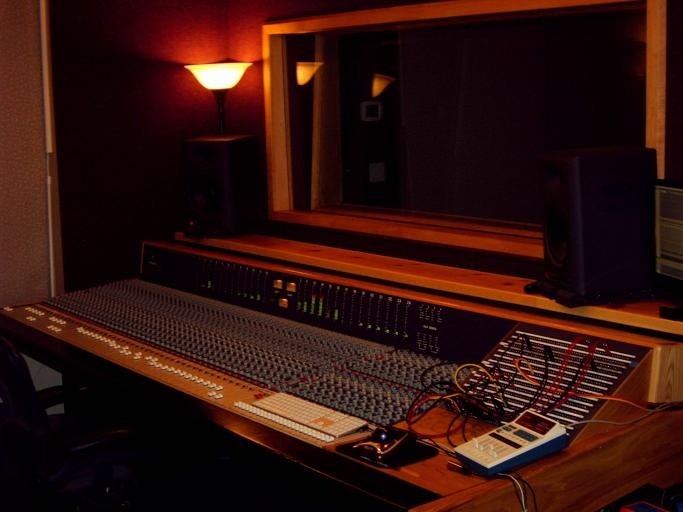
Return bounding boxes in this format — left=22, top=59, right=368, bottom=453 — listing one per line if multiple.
left=294, top=61, right=324, bottom=85
left=183, top=61, right=255, bottom=135
left=372, top=74, right=396, bottom=98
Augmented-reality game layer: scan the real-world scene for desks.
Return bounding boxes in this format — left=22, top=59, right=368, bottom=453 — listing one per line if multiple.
left=3, top=232, right=680, bottom=512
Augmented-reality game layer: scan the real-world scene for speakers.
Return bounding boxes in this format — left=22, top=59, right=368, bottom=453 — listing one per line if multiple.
left=524, top=143, right=657, bottom=306
left=182, top=133, right=257, bottom=239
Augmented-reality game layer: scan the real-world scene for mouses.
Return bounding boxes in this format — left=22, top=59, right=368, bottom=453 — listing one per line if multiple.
left=350, top=427, right=417, bottom=464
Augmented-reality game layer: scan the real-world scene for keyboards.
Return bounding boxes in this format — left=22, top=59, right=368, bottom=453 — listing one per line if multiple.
left=253, top=391, right=368, bottom=439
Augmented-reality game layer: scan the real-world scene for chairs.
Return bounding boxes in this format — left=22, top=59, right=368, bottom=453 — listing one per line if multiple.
left=0, top=338, right=146, bottom=510
